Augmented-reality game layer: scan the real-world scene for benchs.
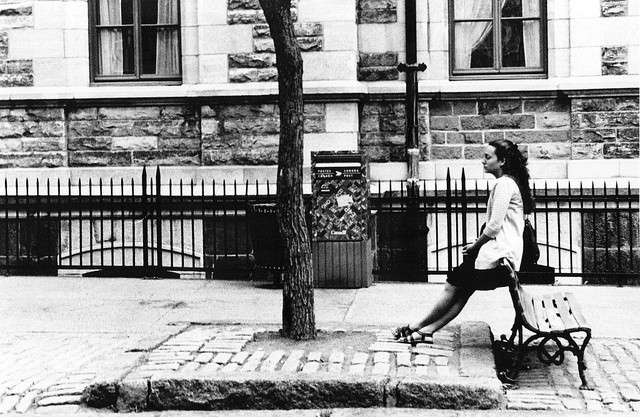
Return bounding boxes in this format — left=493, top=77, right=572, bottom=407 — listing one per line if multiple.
left=499, top=254, right=592, bottom=393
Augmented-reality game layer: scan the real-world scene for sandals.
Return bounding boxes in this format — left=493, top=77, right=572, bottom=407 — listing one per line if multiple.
left=393, top=324, right=419, bottom=340
left=398, top=330, right=434, bottom=344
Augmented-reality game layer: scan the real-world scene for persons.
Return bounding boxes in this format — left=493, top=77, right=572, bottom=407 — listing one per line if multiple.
left=392, top=138, right=535, bottom=343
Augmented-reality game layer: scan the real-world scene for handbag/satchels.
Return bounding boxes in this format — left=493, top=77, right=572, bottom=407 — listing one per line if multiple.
left=480, top=215, right=540, bottom=271
